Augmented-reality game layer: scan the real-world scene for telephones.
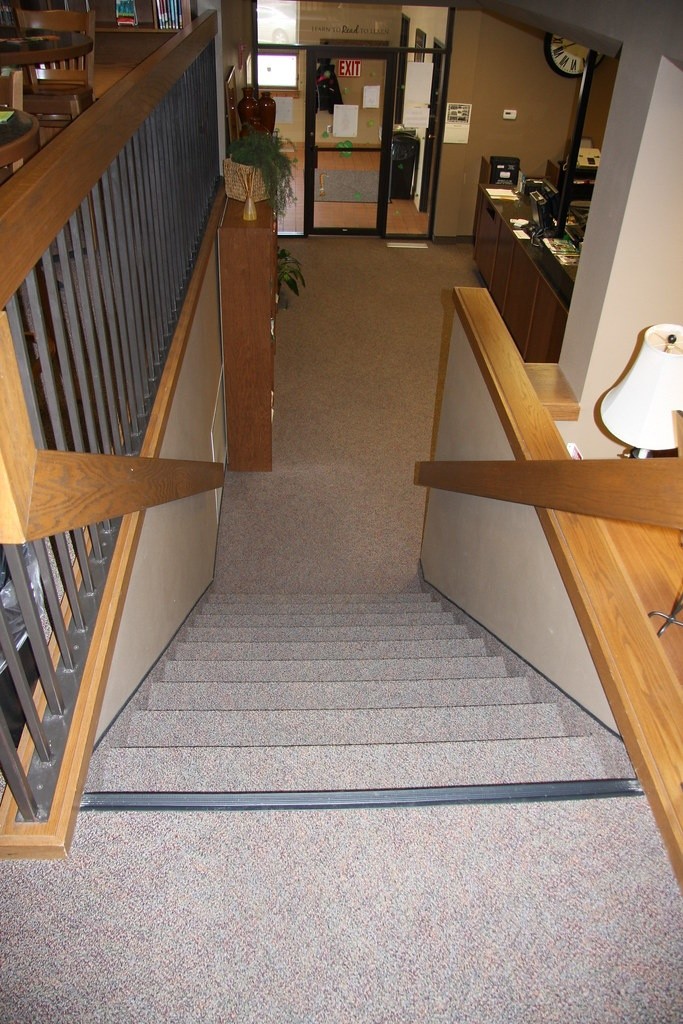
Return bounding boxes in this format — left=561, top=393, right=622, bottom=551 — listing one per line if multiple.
left=566, top=147, right=601, bottom=169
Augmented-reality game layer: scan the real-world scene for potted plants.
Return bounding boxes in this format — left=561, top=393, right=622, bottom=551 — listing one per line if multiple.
left=224, top=123, right=298, bottom=216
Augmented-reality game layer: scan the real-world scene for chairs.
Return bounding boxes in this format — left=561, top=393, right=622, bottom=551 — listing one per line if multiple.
left=15, top=5, right=96, bottom=129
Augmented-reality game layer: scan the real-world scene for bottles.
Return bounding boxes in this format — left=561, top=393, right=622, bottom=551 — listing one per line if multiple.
left=238, top=87, right=276, bottom=140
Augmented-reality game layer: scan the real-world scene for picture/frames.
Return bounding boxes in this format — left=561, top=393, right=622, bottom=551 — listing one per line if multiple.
left=225, top=66, right=239, bottom=145
left=414, top=29, right=426, bottom=62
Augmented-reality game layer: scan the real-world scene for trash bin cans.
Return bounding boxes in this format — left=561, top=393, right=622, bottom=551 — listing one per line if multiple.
left=390, top=132, right=420, bottom=200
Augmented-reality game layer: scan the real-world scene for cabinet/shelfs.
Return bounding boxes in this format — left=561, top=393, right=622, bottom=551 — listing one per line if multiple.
left=217, top=197, right=278, bottom=470
left=473, top=156, right=598, bottom=364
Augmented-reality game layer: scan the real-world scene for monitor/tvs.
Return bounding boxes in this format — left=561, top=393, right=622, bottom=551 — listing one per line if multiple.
left=542, top=178, right=560, bottom=221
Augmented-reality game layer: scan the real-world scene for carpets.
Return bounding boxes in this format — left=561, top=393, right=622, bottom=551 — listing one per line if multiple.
left=315, top=168, right=392, bottom=202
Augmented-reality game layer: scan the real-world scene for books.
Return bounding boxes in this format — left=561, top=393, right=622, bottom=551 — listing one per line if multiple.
left=0, top=111, right=14, bottom=124
left=156, top=0, right=182, bottom=30
left=486, top=189, right=518, bottom=200
left=542, top=238, right=580, bottom=266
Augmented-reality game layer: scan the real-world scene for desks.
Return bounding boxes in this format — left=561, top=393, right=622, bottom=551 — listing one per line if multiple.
left=0, top=105, right=119, bottom=455
left=0, top=27, right=93, bottom=66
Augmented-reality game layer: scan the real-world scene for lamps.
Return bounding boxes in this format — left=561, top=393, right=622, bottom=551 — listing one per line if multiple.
left=600, top=322, right=683, bottom=459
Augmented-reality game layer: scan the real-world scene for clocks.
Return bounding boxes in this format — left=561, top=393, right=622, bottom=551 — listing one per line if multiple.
left=543, top=32, right=606, bottom=78
